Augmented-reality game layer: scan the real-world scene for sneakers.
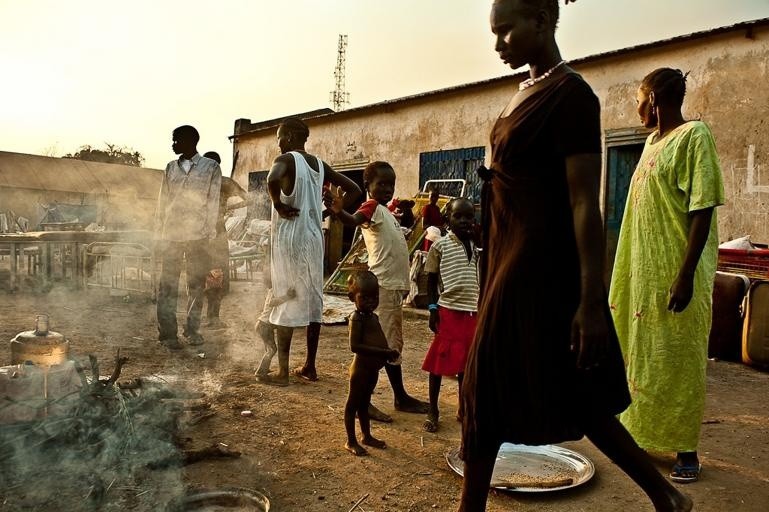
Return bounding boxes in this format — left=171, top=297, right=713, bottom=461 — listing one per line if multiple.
left=162, top=338, right=183, bottom=349
left=184, top=332, right=204, bottom=346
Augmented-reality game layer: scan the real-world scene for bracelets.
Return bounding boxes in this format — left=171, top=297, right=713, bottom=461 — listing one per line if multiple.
left=428, top=303, right=439, bottom=311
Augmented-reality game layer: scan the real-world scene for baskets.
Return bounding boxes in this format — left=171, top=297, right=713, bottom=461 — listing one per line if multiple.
left=717, top=243, right=769, bottom=280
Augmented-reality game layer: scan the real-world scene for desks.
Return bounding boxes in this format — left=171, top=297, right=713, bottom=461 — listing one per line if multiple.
left=0, top=230, right=148, bottom=294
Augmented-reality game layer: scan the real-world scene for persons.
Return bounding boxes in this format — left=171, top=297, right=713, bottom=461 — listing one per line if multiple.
left=261, top=117, right=364, bottom=388
left=152, top=125, right=222, bottom=349
left=456, top=0, right=696, bottom=512
left=322, top=162, right=430, bottom=423
left=202, top=151, right=254, bottom=330
left=423, top=198, right=482, bottom=434
left=422, top=190, right=445, bottom=253
left=343, top=271, right=398, bottom=456
left=254, top=274, right=294, bottom=377
left=608, top=67, right=725, bottom=484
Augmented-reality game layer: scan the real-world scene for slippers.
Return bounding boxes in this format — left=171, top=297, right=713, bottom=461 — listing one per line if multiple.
left=423, top=414, right=437, bottom=432
left=292, top=366, right=319, bottom=381
left=256, top=374, right=289, bottom=386
left=669, top=463, right=702, bottom=483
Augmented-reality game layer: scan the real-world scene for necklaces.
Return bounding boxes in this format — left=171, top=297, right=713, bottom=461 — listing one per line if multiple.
left=518, top=60, right=565, bottom=91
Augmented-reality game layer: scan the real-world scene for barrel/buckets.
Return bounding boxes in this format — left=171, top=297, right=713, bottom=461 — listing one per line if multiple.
left=0, top=315, right=84, bottom=426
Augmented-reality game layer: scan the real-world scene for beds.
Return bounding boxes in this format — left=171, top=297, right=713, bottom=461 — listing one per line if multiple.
left=82, top=242, right=265, bottom=303
